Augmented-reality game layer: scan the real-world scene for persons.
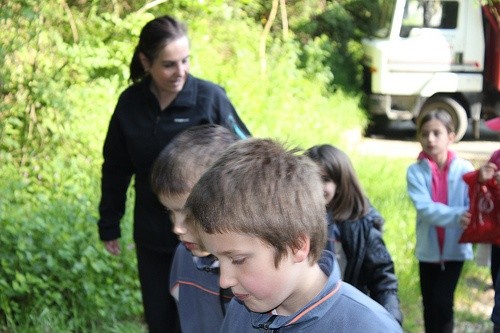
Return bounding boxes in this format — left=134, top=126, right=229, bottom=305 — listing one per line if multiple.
left=302, top=144, right=404, bottom=325
left=478, top=149, right=500, bottom=333
left=407, top=110, right=476, bottom=333
left=150, top=124, right=340, bottom=333
left=97, top=15, right=254, bottom=333
left=186, top=138, right=404, bottom=333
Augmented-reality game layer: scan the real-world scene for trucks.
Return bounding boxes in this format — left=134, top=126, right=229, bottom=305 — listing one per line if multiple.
left=363, top=0, right=500, bottom=144
